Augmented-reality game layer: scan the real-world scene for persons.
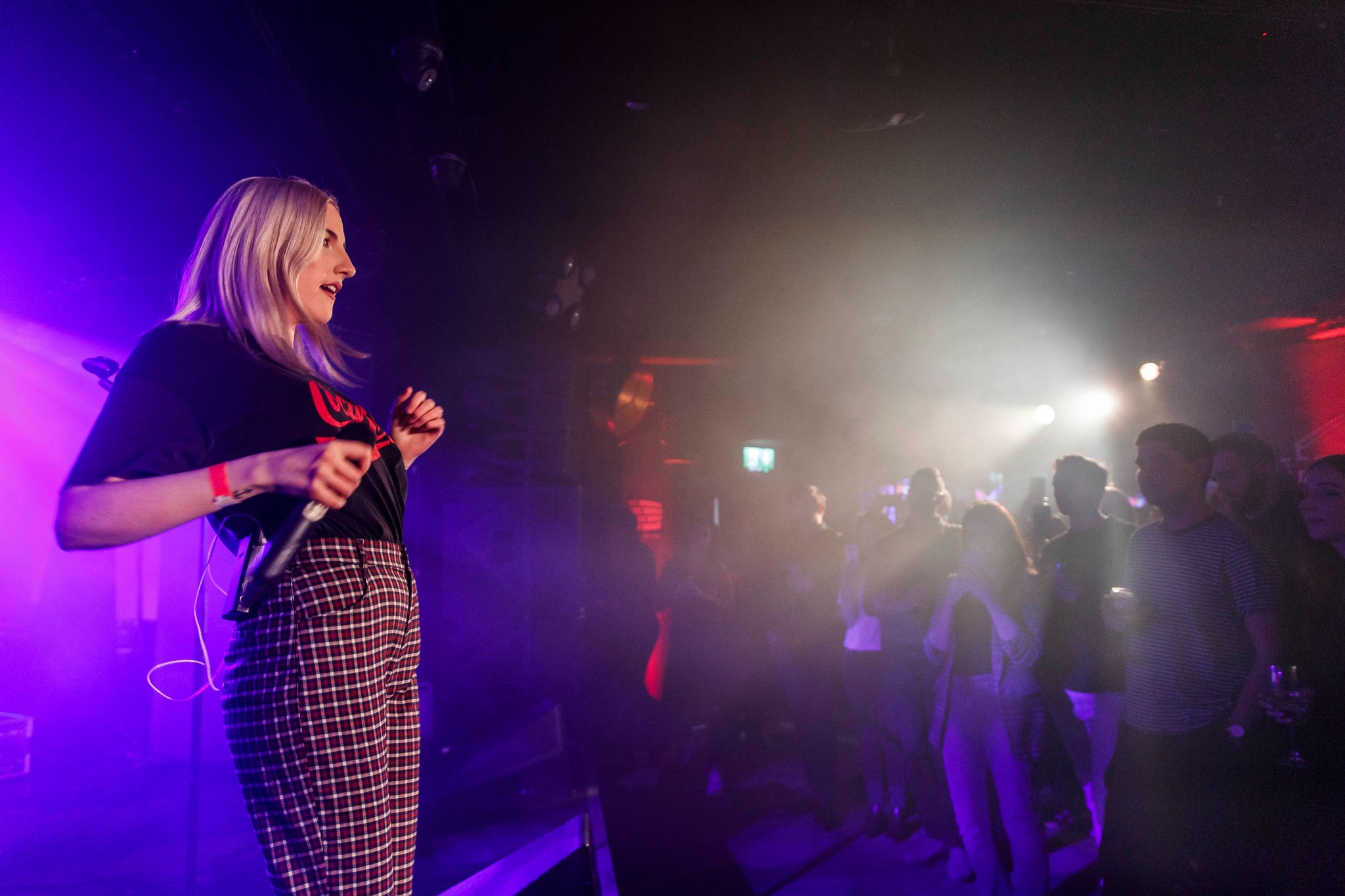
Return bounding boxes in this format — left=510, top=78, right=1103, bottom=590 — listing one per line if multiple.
left=57, top=176, right=444, bottom=896
left=628, top=423, right=1345, bottom=896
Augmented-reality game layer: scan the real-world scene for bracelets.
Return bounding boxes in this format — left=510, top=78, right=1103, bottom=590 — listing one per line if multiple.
left=209, top=462, right=236, bottom=507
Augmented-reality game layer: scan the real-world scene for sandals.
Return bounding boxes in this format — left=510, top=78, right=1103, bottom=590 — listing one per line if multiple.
left=948, top=845, right=972, bottom=883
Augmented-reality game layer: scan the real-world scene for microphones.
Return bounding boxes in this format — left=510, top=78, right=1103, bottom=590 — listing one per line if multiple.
left=240, top=423, right=376, bottom=609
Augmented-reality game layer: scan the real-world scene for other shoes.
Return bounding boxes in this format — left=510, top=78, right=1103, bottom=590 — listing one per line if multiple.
left=865, top=800, right=885, bottom=837
left=889, top=805, right=906, bottom=839
left=904, top=837, right=946, bottom=863
